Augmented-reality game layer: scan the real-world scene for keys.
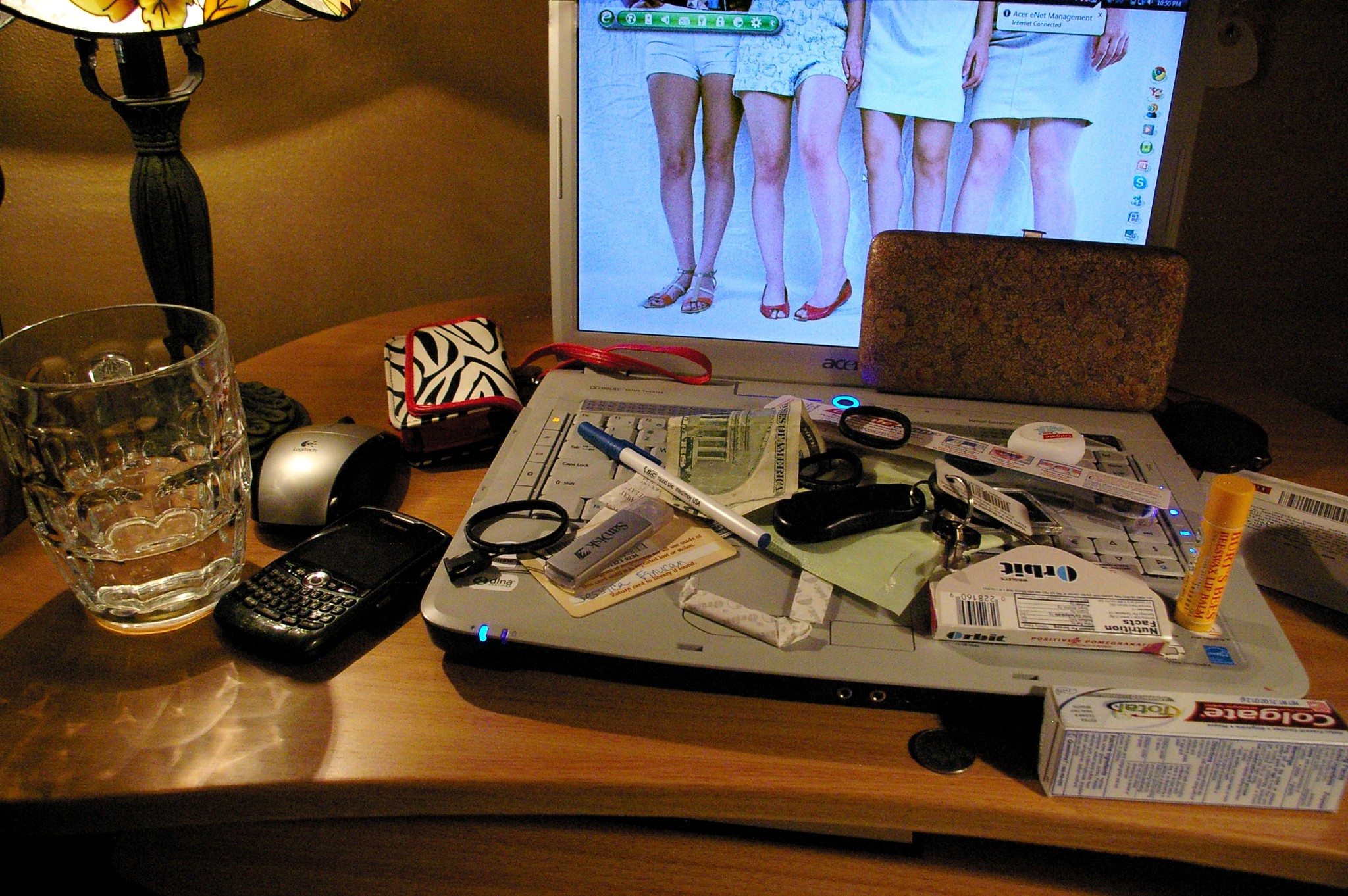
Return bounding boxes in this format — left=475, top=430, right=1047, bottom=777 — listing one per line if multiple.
left=926, top=471, right=1037, bottom=545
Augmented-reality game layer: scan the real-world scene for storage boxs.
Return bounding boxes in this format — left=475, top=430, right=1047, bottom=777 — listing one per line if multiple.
left=1036, top=688, right=1347, bottom=818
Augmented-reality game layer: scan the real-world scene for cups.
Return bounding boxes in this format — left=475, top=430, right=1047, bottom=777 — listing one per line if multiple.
left=1, top=303, right=251, bottom=635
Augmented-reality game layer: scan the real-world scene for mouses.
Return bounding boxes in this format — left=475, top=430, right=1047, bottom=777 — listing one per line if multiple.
left=248, top=416, right=399, bottom=526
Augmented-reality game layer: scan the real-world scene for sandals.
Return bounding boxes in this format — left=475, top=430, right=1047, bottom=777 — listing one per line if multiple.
left=681, top=269, right=718, bottom=314
left=644, top=264, right=697, bottom=308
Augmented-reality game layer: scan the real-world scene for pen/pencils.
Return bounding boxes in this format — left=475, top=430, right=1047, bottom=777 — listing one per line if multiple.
left=578, top=421, right=772, bottom=548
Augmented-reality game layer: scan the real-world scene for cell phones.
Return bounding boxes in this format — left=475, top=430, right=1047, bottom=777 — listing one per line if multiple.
left=210, top=505, right=454, bottom=663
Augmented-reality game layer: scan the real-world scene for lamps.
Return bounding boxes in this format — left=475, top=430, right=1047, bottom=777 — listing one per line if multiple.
left=0, top=0, right=360, bottom=483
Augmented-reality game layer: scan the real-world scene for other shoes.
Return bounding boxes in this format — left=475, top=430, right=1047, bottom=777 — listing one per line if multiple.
left=793, top=279, right=852, bottom=322
left=760, top=283, right=789, bottom=319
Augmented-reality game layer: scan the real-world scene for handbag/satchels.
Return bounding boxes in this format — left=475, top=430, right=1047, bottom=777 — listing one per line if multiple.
left=383, top=314, right=524, bottom=469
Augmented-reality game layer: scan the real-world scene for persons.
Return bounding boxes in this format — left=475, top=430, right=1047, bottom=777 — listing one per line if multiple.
left=854, top=0, right=997, bottom=239
left=621, top=0, right=754, bottom=315
left=731, top=0, right=865, bottom=321
left=951, top=0, right=1132, bottom=243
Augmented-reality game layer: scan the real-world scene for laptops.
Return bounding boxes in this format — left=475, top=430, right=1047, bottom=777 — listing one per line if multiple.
left=419, top=1, right=1312, bottom=719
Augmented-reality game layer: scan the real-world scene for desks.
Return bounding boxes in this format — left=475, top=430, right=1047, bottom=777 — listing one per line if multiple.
left=0, top=287, right=1346, bottom=896
